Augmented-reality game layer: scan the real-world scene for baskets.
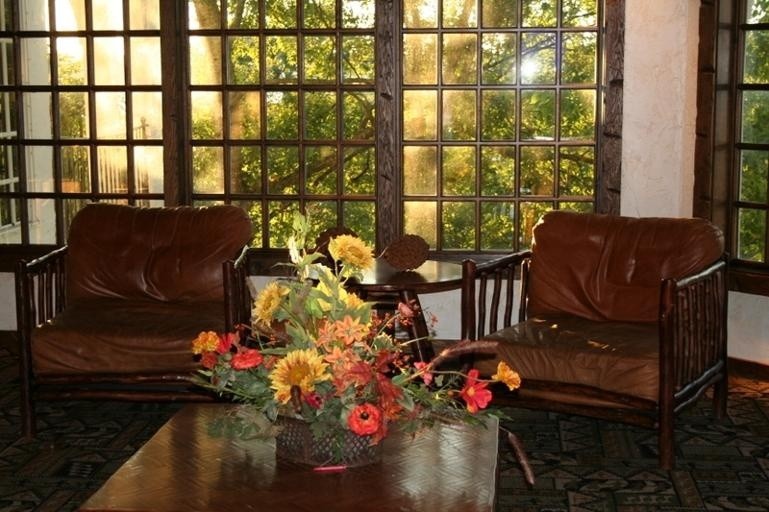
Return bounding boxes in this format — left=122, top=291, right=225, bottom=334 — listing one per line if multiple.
left=276, top=414, right=384, bottom=468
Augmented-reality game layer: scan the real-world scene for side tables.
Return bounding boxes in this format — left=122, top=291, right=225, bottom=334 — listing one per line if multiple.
left=309, top=258, right=464, bottom=379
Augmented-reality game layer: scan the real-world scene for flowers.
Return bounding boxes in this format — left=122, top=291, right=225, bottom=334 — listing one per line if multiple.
left=189, top=209, right=537, bottom=489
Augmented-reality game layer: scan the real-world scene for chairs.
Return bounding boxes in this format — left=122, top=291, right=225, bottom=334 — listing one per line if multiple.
left=461, top=205, right=733, bottom=471
left=13, top=198, right=257, bottom=441
